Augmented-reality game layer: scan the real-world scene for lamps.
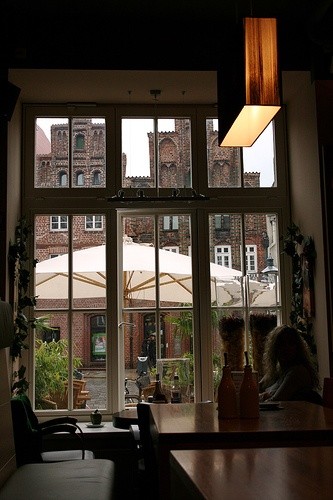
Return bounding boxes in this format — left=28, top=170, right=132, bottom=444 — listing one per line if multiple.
left=217, top=17, right=283, bottom=147
left=260, top=258, right=279, bottom=306
left=106, top=90, right=208, bottom=201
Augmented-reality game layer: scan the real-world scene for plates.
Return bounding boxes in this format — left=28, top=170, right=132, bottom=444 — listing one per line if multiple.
left=86, top=423, right=105, bottom=427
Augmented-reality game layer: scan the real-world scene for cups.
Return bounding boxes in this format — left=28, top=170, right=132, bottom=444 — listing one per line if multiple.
left=90, top=412, right=102, bottom=425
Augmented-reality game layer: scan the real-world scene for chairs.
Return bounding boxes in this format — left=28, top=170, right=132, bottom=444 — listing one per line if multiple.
left=124, top=378, right=141, bottom=403
left=49, top=380, right=86, bottom=408
left=10, top=394, right=94, bottom=465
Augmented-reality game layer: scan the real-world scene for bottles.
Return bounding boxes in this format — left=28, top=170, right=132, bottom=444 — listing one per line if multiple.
left=153, top=374, right=164, bottom=399
left=239, top=351, right=259, bottom=419
left=217, top=353, right=239, bottom=419
left=171, top=375, right=182, bottom=403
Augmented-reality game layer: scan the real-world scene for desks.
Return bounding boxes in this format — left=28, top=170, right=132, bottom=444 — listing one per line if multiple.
left=170, top=446, right=333, bottom=500
left=46, top=421, right=134, bottom=459
left=80, top=390, right=90, bottom=394
left=78, top=395, right=91, bottom=400
left=112, top=404, right=333, bottom=458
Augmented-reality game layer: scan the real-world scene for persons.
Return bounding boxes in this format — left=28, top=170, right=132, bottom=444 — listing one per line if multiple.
left=96, top=337, right=104, bottom=346
left=148, top=335, right=156, bottom=367
left=258, top=326, right=323, bottom=406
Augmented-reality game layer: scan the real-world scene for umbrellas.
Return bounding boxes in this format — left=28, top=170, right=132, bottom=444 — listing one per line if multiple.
left=216, top=279, right=279, bottom=350
left=36, top=240, right=244, bottom=323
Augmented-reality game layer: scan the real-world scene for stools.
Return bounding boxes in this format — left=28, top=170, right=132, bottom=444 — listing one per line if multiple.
left=0, top=460, right=115, bottom=500
